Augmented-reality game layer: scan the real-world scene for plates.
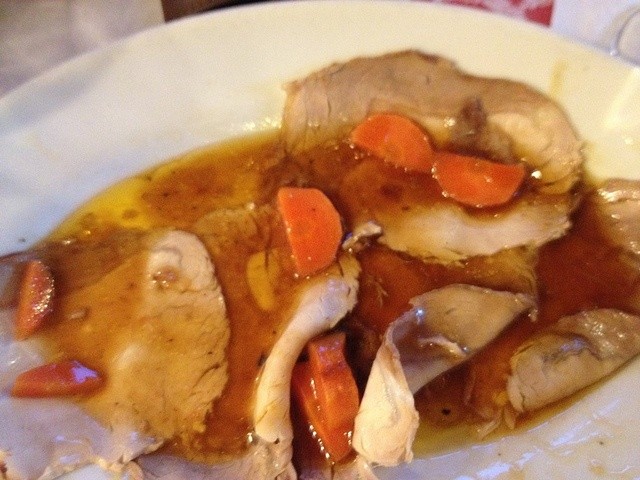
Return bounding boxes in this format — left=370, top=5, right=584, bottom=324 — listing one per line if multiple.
left=0, top=0, right=640, bottom=480
